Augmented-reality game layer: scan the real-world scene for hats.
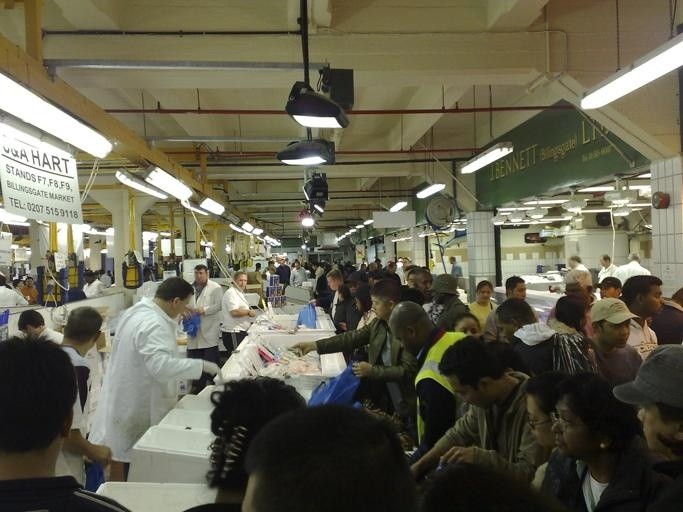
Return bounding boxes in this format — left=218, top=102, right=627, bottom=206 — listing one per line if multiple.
left=427, top=274, right=460, bottom=296
left=589, top=298, right=640, bottom=326
left=611, top=343, right=683, bottom=406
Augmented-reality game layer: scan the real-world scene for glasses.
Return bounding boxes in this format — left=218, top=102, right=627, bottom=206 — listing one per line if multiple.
left=547, top=413, right=585, bottom=431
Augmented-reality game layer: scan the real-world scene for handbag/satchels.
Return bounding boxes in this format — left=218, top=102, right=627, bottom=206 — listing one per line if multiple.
left=298, top=303, right=318, bottom=328
left=307, top=363, right=361, bottom=408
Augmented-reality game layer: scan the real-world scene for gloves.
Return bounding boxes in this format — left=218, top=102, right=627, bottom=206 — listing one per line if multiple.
left=203, top=361, right=223, bottom=380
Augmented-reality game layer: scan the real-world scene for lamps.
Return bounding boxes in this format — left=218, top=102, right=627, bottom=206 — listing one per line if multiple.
left=392, top=232, right=413, bottom=243
left=491, top=173, right=651, bottom=239
left=418, top=219, right=467, bottom=238
left=142, top=165, right=193, bottom=202
left=580, top=2, right=683, bottom=110
left=277, top=128, right=330, bottom=166
left=335, top=210, right=374, bottom=242
left=81, top=224, right=114, bottom=235
left=1, top=207, right=31, bottom=227
left=389, top=178, right=408, bottom=213
left=198, top=196, right=224, bottom=215
left=416, top=126, right=445, bottom=198
left=461, top=85, right=513, bottom=173
left=143, top=230, right=171, bottom=241
left=285, top=1, right=349, bottom=129
left=180, top=199, right=210, bottom=216
left=229, top=221, right=281, bottom=246
left=115, top=168, right=168, bottom=200
left=0, top=72, right=113, bottom=159
left=299, top=174, right=328, bottom=226
